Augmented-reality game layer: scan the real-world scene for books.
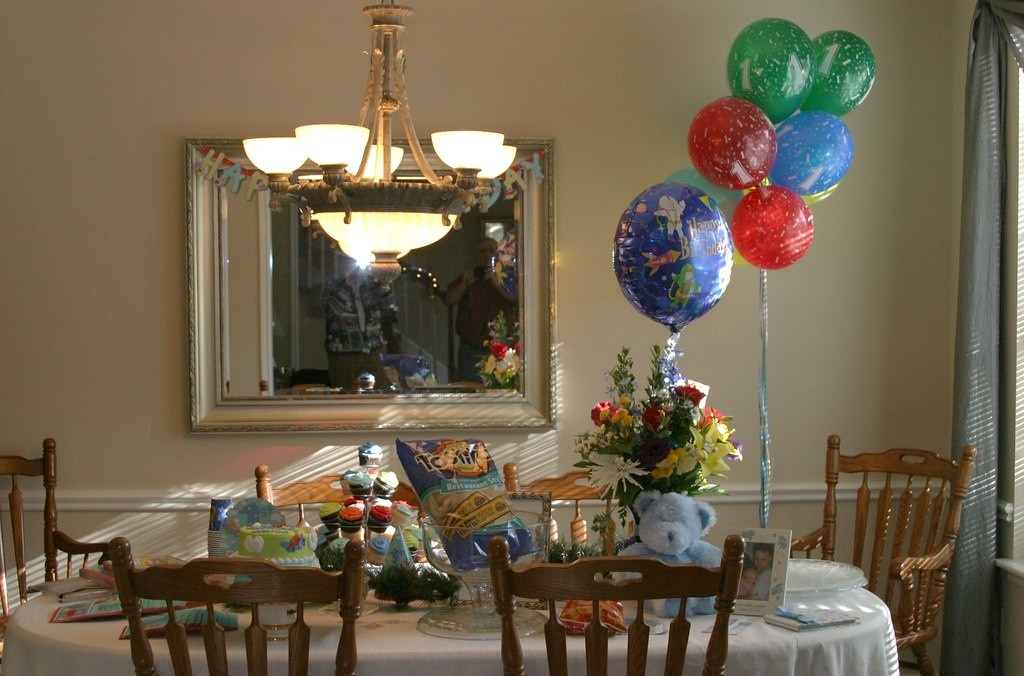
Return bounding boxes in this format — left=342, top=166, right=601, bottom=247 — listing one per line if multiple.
left=765, top=604, right=859, bottom=631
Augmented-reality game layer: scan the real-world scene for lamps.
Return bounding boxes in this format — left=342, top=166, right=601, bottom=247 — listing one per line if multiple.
left=242, top=0, right=512, bottom=281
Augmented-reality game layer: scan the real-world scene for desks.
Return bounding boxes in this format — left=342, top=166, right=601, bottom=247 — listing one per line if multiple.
left=3, top=580, right=901, bottom=676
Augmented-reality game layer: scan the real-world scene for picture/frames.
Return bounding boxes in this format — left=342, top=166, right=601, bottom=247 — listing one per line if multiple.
left=731, top=527, right=793, bottom=614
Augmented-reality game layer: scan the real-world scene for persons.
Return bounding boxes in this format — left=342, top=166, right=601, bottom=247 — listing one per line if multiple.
left=737, top=568, right=757, bottom=602
left=442, top=238, right=519, bottom=383
left=750, top=547, right=772, bottom=601
left=323, top=259, right=398, bottom=387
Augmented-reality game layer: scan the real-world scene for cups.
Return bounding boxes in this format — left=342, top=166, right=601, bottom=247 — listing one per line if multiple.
left=208, top=497, right=233, bottom=558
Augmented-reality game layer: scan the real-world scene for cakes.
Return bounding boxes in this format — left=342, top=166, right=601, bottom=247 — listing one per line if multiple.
left=227, top=527, right=320, bottom=608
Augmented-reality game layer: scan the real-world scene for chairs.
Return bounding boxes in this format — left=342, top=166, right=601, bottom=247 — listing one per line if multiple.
left=0, top=438, right=110, bottom=655
left=488, top=534, right=745, bottom=676
left=110, top=536, right=365, bottom=676
left=790, top=433, right=977, bottom=676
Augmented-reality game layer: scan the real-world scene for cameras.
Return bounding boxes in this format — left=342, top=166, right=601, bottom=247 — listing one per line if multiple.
left=473, top=265, right=489, bottom=278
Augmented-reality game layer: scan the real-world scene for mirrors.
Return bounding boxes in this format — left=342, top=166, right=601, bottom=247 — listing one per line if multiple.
left=186, top=137, right=558, bottom=433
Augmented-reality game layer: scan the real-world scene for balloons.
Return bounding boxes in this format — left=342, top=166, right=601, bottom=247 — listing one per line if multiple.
left=610, top=17, right=876, bottom=331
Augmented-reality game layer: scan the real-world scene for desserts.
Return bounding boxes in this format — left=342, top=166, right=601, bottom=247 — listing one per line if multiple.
left=355, top=372, right=375, bottom=390
left=316, top=440, right=424, bottom=565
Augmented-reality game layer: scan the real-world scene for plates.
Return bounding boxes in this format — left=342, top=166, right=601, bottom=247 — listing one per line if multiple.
left=785, top=558, right=868, bottom=600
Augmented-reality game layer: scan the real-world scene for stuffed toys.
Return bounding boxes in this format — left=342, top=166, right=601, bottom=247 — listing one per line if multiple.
left=612, top=490, right=724, bottom=619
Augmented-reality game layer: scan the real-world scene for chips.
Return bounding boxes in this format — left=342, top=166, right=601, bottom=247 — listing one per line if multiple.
left=564, top=600, right=620, bottom=625
